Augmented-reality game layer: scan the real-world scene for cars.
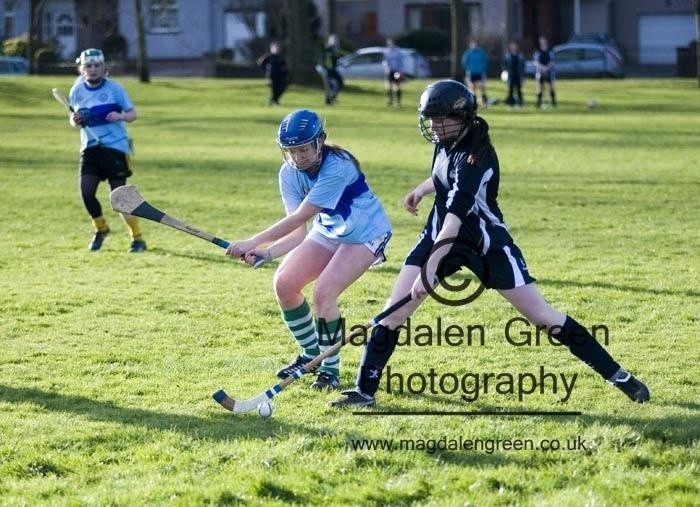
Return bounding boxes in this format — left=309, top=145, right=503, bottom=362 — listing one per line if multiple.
left=0, top=57, right=29, bottom=74
left=315, top=43, right=431, bottom=78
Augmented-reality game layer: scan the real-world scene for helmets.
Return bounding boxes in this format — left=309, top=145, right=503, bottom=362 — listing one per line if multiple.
left=75, top=48, right=105, bottom=83
left=276, top=108, right=322, bottom=172
left=418, top=79, right=478, bottom=141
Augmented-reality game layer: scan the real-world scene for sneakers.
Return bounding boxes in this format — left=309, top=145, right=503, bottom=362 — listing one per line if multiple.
left=309, top=371, right=340, bottom=391
left=88, top=227, right=110, bottom=251
left=612, top=366, right=649, bottom=403
left=325, top=389, right=376, bottom=409
left=276, top=354, right=321, bottom=378
left=126, top=239, right=146, bottom=252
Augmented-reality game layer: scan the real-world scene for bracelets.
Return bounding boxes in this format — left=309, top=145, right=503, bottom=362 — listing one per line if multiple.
left=267, top=246, right=272, bottom=258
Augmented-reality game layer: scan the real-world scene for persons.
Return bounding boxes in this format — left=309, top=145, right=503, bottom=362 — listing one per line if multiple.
left=67, top=47, right=146, bottom=254
left=532, top=31, right=557, bottom=107
left=461, top=36, right=491, bottom=109
left=223, top=108, right=393, bottom=389
left=381, top=35, right=402, bottom=108
left=500, top=39, right=526, bottom=105
left=318, top=30, right=346, bottom=104
left=257, top=38, right=289, bottom=108
left=323, top=79, right=651, bottom=408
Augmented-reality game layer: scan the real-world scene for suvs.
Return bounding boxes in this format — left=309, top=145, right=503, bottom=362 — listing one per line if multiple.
left=500, top=33, right=625, bottom=79
